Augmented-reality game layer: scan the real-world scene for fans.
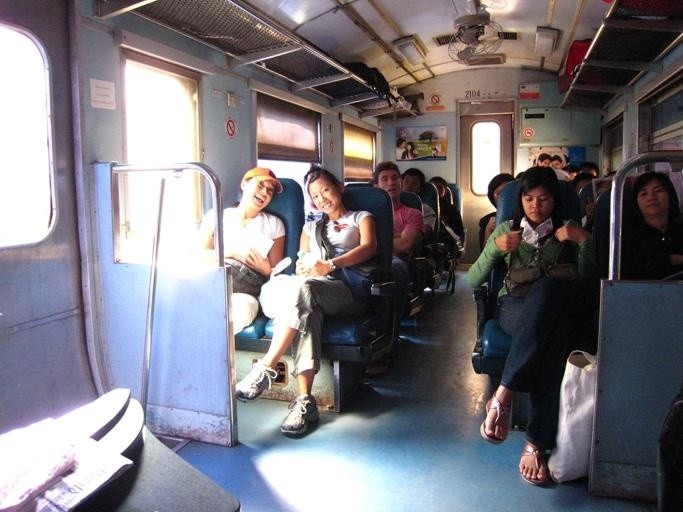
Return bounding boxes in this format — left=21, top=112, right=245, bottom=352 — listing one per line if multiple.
left=447, top=15, right=504, bottom=65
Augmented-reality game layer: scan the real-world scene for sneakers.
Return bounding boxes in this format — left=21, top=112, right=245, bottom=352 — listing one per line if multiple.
left=280, top=395, right=320, bottom=437
left=234, top=359, right=280, bottom=404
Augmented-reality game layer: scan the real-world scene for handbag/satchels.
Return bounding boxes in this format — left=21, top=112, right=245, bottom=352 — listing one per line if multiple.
left=546, top=350, right=598, bottom=484
left=331, top=252, right=382, bottom=300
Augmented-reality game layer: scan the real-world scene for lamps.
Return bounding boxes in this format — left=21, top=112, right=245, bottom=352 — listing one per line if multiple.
left=393, top=36, right=427, bottom=67
left=534, top=27, right=559, bottom=57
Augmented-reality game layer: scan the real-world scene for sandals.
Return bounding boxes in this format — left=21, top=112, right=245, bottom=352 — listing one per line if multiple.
left=519, top=440, right=549, bottom=486
left=479, top=390, right=512, bottom=446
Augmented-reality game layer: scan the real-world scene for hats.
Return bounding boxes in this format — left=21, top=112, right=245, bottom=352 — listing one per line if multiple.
left=243, top=167, right=283, bottom=195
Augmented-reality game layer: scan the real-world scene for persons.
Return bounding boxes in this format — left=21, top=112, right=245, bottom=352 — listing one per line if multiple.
left=394, top=137, right=418, bottom=160
left=401, top=142, right=417, bottom=159
left=198, top=161, right=467, bottom=435
left=468, top=154, right=683, bottom=486
